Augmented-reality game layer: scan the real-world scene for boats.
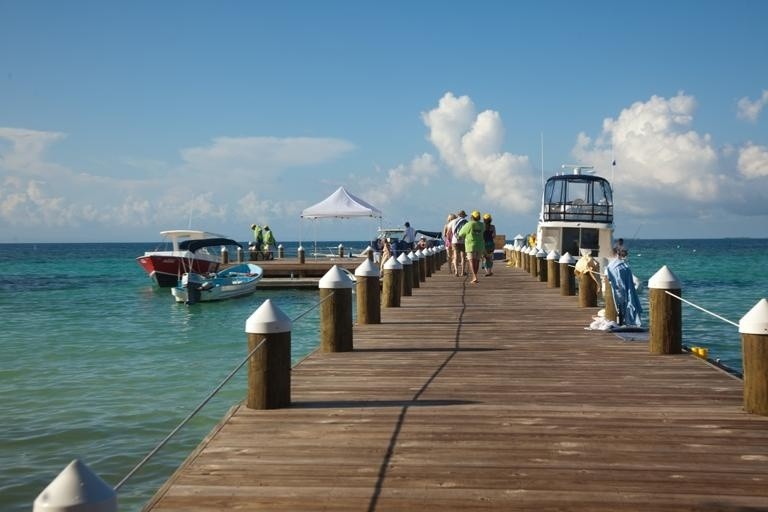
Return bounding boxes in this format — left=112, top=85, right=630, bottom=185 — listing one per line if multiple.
left=537, top=132, right=641, bottom=294
left=137, top=229, right=263, bottom=303
left=313, top=228, right=415, bottom=258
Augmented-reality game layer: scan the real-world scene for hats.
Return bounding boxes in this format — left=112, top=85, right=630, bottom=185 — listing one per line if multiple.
left=471, top=211, right=480, bottom=218
left=483, top=214, right=491, bottom=217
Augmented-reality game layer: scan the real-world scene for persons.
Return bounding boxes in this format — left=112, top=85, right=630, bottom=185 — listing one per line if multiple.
left=250, top=224, right=266, bottom=261
left=248, top=241, right=259, bottom=261
left=441, top=211, right=497, bottom=283
left=528, top=232, right=536, bottom=249
left=400, top=221, right=416, bottom=250
left=614, top=239, right=629, bottom=258
left=262, top=225, right=279, bottom=260
left=416, top=234, right=444, bottom=250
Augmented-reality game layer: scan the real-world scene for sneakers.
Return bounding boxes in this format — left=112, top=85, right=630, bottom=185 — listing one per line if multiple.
left=455, top=272, right=466, bottom=276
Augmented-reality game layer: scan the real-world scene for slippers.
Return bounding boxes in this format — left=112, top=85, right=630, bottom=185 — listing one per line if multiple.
left=485, top=271, right=493, bottom=276
left=472, top=279, right=478, bottom=283
